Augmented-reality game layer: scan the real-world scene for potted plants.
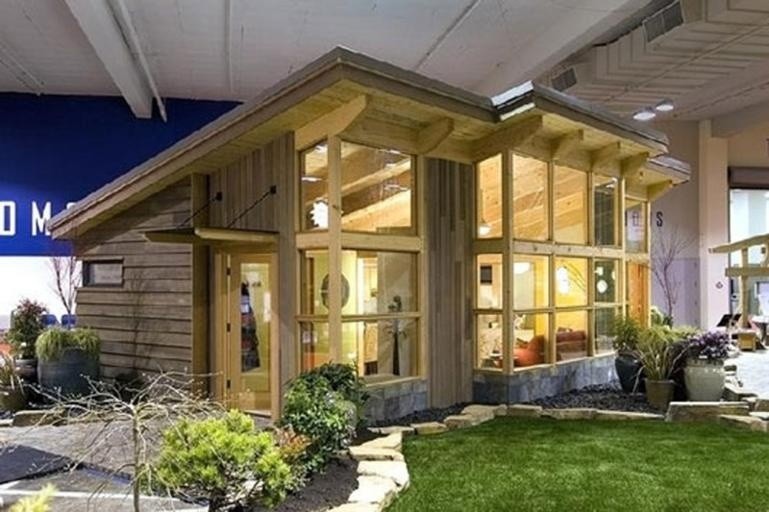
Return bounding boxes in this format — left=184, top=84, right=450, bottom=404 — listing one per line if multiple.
left=0, top=296, right=101, bottom=413
left=605, top=308, right=684, bottom=411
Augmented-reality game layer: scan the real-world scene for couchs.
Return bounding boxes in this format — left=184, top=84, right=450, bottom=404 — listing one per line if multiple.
left=517, top=329, right=588, bottom=368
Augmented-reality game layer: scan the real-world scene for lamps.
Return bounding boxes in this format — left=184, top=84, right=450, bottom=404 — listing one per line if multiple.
left=632, top=97, right=677, bottom=124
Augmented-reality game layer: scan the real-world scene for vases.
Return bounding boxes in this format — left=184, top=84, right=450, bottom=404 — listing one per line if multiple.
left=683, top=359, right=728, bottom=402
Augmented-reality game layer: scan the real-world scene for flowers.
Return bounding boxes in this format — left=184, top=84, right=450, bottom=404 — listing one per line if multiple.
left=680, top=328, right=732, bottom=366
